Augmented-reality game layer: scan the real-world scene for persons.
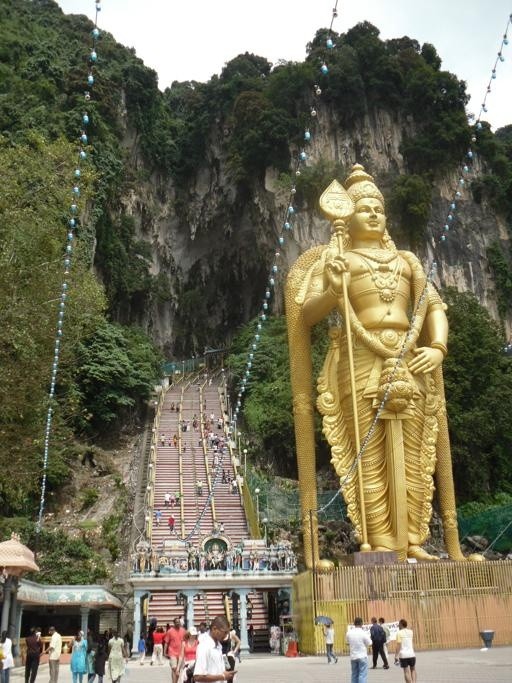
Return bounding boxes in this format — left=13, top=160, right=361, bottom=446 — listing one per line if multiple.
left=154, top=364, right=255, bottom=611
left=0, top=615, right=241, bottom=682
left=270, top=622, right=280, bottom=654
left=301, top=164, right=449, bottom=564
left=322, top=617, right=417, bottom=683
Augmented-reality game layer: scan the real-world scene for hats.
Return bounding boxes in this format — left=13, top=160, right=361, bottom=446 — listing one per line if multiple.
left=186, top=625, right=199, bottom=636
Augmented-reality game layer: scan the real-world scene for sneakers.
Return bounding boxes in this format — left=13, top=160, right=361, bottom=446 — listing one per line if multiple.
left=328, top=655, right=339, bottom=664
left=373, top=664, right=389, bottom=668
left=150, top=660, right=154, bottom=666
